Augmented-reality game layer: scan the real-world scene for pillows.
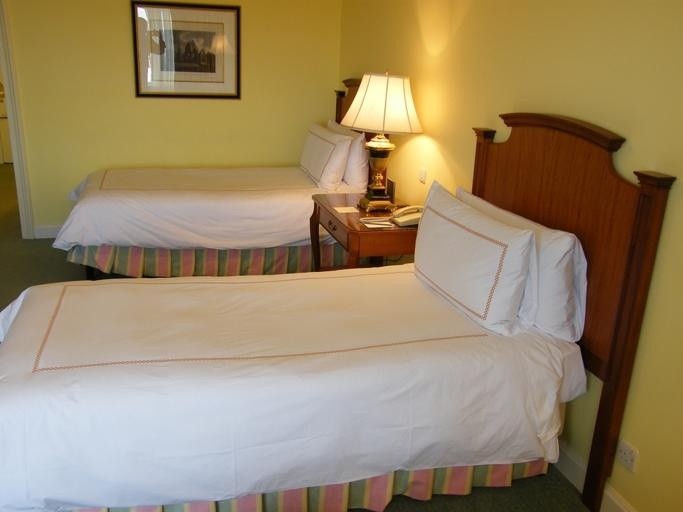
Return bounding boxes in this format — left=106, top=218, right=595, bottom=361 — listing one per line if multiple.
left=455, top=185, right=588, bottom=342
left=299, top=123, right=353, bottom=193
left=327, top=118, right=369, bottom=193
left=414, top=180, right=533, bottom=338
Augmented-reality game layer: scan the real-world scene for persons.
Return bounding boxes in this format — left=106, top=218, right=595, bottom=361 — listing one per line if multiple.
left=136, top=17, right=167, bottom=92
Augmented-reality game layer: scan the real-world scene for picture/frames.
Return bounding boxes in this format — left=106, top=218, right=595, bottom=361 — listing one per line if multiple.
left=131, top=0, right=240, bottom=98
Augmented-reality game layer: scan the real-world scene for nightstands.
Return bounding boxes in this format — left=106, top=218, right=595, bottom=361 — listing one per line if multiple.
left=310, top=193, right=418, bottom=272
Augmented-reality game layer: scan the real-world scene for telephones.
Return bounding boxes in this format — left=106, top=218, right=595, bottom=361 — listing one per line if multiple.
left=390, top=205, right=424, bottom=227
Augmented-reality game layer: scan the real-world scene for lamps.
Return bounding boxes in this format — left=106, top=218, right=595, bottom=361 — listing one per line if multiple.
left=340, top=73, right=423, bottom=212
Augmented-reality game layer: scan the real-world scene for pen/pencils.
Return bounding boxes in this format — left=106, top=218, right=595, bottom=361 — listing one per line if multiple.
left=361, top=219, right=392, bottom=226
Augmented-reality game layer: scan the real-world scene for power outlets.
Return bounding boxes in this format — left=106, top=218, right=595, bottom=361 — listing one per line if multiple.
left=616, top=441, right=636, bottom=474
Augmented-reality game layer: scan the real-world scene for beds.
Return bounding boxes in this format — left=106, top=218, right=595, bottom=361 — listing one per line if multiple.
left=79, top=79, right=392, bottom=280
left=0, top=112, right=676, bottom=512
